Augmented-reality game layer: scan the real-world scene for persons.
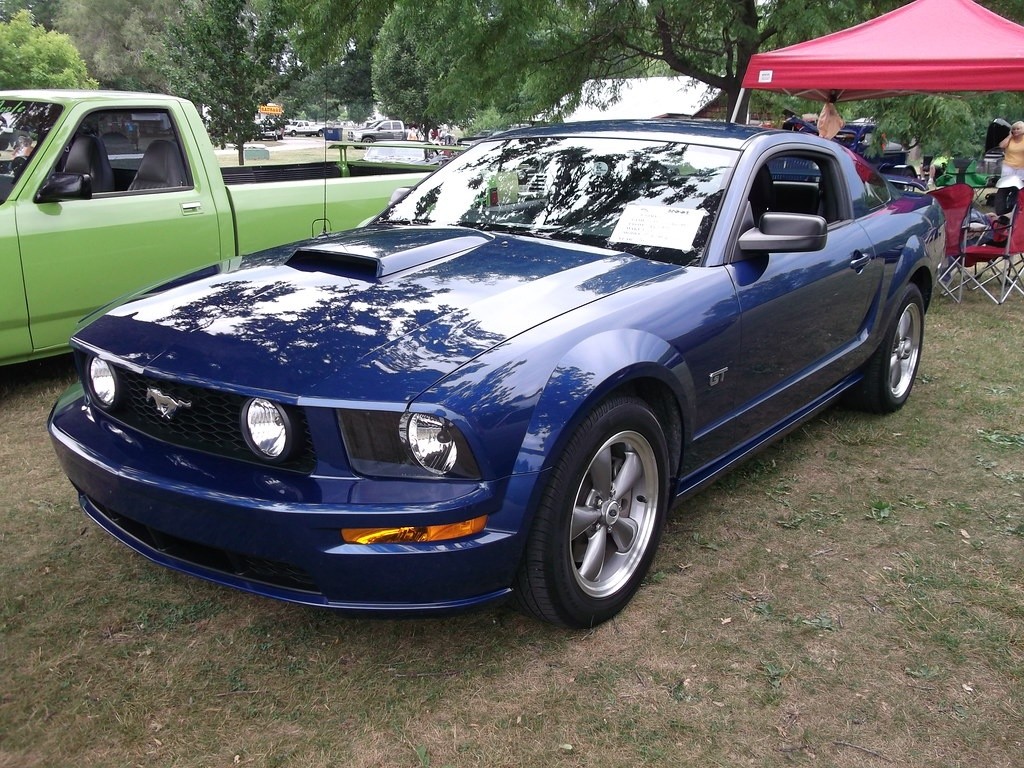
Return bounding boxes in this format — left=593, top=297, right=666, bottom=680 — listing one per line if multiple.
left=919, top=154, right=954, bottom=186
left=128, top=121, right=139, bottom=147
left=999, top=120, right=1024, bottom=183
left=431, top=127, right=444, bottom=141
left=10, top=136, right=31, bottom=154
left=937, top=209, right=1012, bottom=283
left=406, top=127, right=418, bottom=139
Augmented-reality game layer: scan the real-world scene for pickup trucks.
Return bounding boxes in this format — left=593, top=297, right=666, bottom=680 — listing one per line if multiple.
left=255, top=119, right=412, bottom=150
left=0, top=88, right=472, bottom=367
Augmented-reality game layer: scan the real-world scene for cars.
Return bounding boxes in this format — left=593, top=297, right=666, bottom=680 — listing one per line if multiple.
left=46, top=118, right=946, bottom=632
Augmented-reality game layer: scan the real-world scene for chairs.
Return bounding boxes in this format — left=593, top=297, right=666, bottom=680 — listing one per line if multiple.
left=61, top=133, right=116, bottom=192
left=934, top=159, right=958, bottom=187
left=923, top=181, right=976, bottom=304
left=748, top=161, right=774, bottom=225
left=959, top=188, right=1024, bottom=306
left=127, top=137, right=189, bottom=190
left=964, top=159, right=991, bottom=207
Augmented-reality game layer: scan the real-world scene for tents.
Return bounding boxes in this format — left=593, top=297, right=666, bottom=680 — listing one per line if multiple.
left=729, top=1, right=1024, bottom=123
